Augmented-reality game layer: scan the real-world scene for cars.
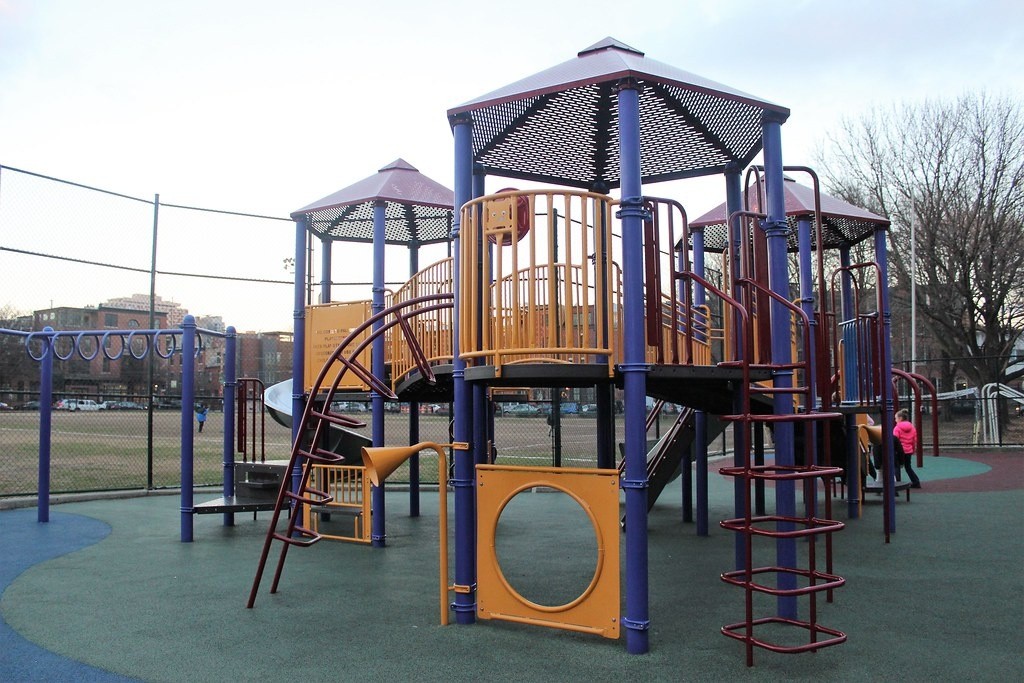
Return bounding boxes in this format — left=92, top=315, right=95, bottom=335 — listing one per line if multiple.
left=0, top=397, right=685, bottom=413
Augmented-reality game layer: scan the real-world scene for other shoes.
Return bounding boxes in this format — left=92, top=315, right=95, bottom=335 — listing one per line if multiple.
left=911, top=482, right=921, bottom=489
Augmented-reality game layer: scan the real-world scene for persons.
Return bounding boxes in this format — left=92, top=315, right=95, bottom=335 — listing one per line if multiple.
left=832, top=391, right=876, bottom=481
left=196, top=403, right=209, bottom=433
left=893, top=408, right=921, bottom=488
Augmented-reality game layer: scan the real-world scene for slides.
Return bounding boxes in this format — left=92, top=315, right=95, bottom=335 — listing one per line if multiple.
left=258, top=374, right=373, bottom=484
left=619, top=406, right=736, bottom=496
